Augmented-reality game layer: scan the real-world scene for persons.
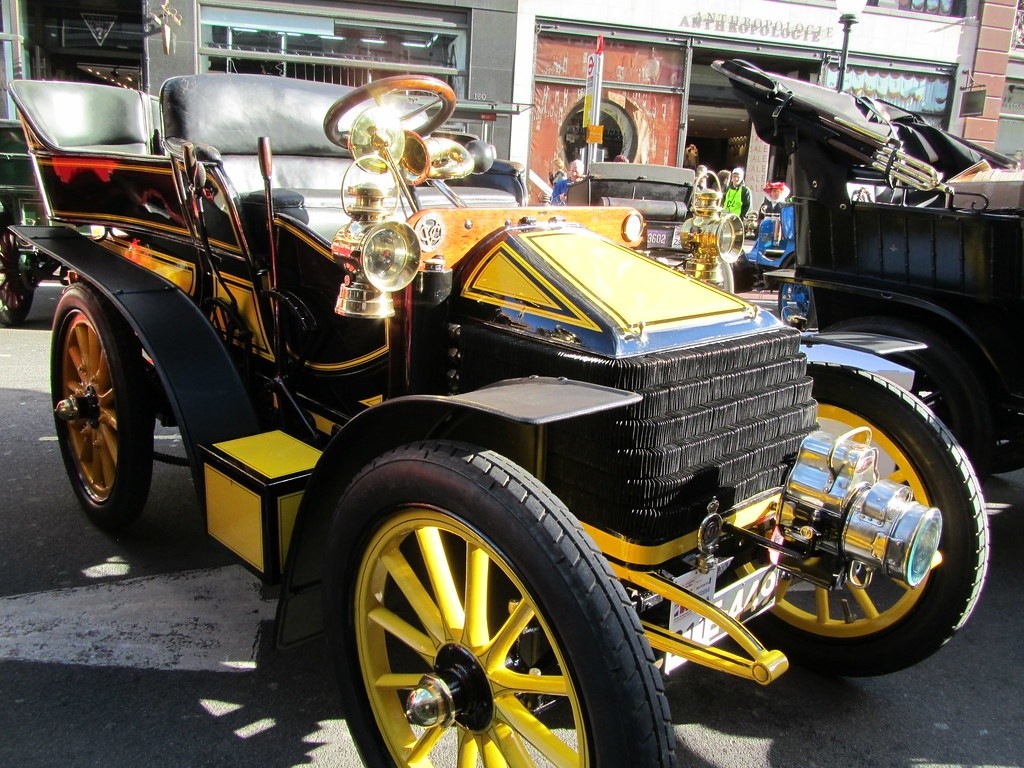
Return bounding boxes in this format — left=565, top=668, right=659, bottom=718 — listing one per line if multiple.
left=696, top=165, right=750, bottom=221
left=548, top=158, right=585, bottom=207
left=614, top=155, right=629, bottom=163
left=759, top=181, right=790, bottom=219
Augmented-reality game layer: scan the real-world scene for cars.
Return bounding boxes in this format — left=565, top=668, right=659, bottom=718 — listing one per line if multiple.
left=7, top=73, right=991, bottom=768
left=710, top=55, right=1024, bottom=475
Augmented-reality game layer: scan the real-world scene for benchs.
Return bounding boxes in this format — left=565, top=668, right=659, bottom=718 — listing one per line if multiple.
left=6, top=76, right=168, bottom=166
left=566, top=162, right=695, bottom=220
left=160, top=73, right=528, bottom=241
left=710, top=58, right=1019, bottom=206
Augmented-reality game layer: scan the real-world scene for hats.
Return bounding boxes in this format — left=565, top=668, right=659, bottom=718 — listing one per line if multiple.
left=763, top=183, right=784, bottom=193
left=732, top=168, right=744, bottom=176
